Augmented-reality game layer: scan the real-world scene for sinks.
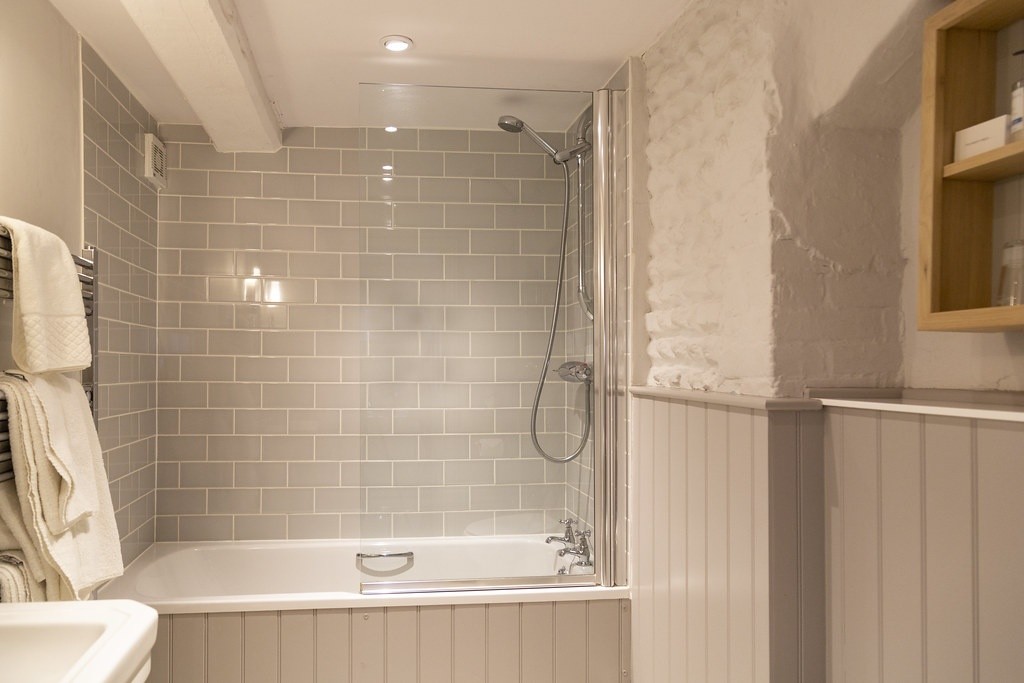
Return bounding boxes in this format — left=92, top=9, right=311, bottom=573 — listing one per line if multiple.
left=1, top=599, right=159, bottom=683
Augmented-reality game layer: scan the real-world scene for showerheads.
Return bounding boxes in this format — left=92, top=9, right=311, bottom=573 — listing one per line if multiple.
left=498, top=116, right=556, bottom=158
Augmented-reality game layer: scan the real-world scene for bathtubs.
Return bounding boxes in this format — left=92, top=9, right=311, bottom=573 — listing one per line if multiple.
left=92, top=538, right=630, bottom=680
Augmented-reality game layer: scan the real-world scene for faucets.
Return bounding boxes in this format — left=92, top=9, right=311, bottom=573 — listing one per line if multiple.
left=558, top=529, right=591, bottom=557
left=545, top=517, right=574, bottom=544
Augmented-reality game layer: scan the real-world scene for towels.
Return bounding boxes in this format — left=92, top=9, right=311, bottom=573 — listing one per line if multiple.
left=0, top=373, right=124, bottom=605
left=1, top=215, right=91, bottom=373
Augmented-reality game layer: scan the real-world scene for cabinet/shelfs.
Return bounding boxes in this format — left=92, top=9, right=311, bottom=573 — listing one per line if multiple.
left=920, top=0, right=1024, bottom=332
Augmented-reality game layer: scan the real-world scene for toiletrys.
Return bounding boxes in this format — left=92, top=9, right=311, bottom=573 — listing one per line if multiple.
left=1011, top=51, right=1024, bottom=142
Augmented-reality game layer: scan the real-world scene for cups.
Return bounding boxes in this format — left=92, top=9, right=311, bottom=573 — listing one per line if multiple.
left=999, top=240, right=1024, bottom=309
